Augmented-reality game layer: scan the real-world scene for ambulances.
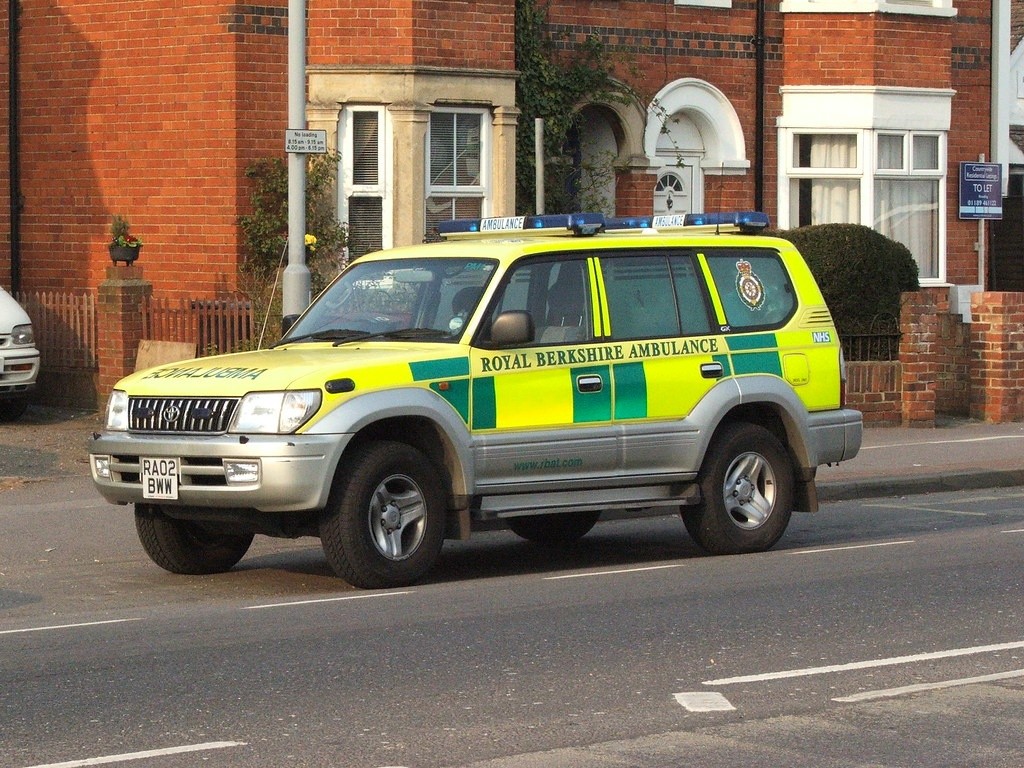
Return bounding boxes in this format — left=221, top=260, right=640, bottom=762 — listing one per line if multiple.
left=88, top=206, right=869, bottom=590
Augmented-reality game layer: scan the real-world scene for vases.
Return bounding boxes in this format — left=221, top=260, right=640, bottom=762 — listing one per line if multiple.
left=110, top=246, right=139, bottom=266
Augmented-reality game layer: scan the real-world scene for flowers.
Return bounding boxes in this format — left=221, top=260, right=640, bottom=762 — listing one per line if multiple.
left=108, top=212, right=141, bottom=247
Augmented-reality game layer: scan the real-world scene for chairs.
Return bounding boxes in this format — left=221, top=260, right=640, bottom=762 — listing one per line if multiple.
left=539, top=280, right=587, bottom=343
left=451, top=286, right=491, bottom=345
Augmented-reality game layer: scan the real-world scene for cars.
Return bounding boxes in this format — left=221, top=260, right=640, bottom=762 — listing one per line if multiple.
left=0, top=282, right=40, bottom=420
